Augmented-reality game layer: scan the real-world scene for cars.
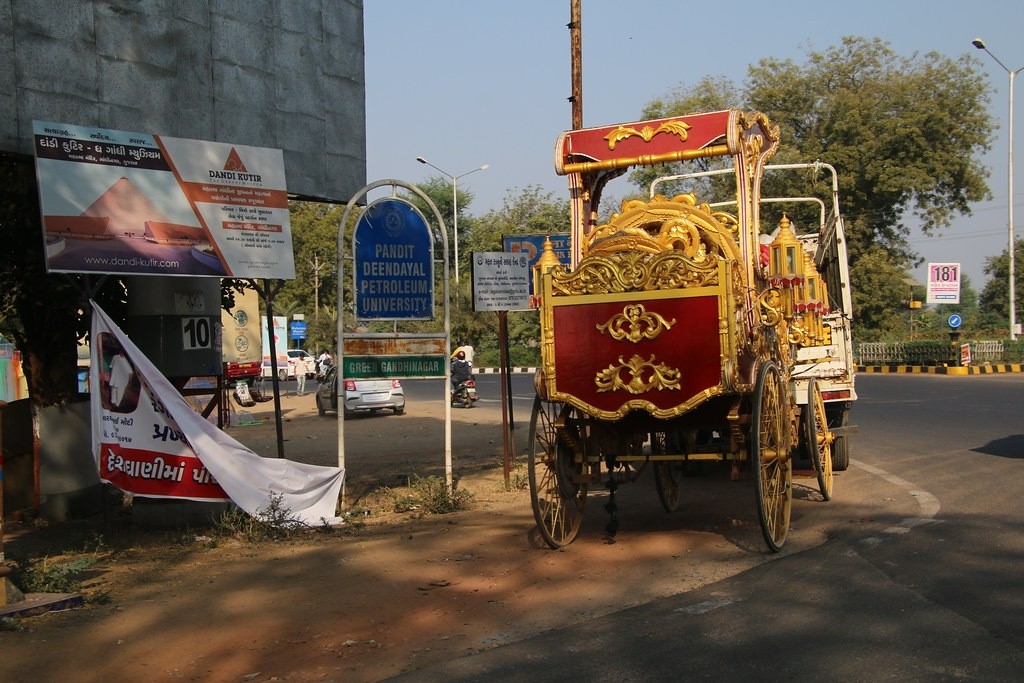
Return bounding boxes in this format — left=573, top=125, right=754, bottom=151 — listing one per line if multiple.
left=316, top=365, right=405, bottom=420
left=257, top=350, right=320, bottom=382
left=223, top=360, right=262, bottom=387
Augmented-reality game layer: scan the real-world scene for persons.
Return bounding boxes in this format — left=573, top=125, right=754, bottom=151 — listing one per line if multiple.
left=450, top=340, right=474, bottom=394
left=315, top=349, right=333, bottom=377
left=294, top=352, right=311, bottom=396
left=108, top=350, right=133, bottom=410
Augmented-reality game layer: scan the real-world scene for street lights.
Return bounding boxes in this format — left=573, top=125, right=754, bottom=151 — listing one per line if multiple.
left=971, top=38, right=1024, bottom=342
left=415, top=156, right=491, bottom=310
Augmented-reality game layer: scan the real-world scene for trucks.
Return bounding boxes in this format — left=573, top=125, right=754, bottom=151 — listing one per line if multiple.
left=648, top=161, right=859, bottom=471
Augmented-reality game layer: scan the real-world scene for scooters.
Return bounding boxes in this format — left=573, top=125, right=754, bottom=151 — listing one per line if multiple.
left=451, top=374, right=481, bottom=408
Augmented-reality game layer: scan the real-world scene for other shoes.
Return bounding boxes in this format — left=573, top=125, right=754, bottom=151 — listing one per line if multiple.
left=451, top=390, right=455, bottom=394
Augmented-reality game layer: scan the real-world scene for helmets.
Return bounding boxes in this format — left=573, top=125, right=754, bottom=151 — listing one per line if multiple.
left=458, top=351, right=465, bottom=356
